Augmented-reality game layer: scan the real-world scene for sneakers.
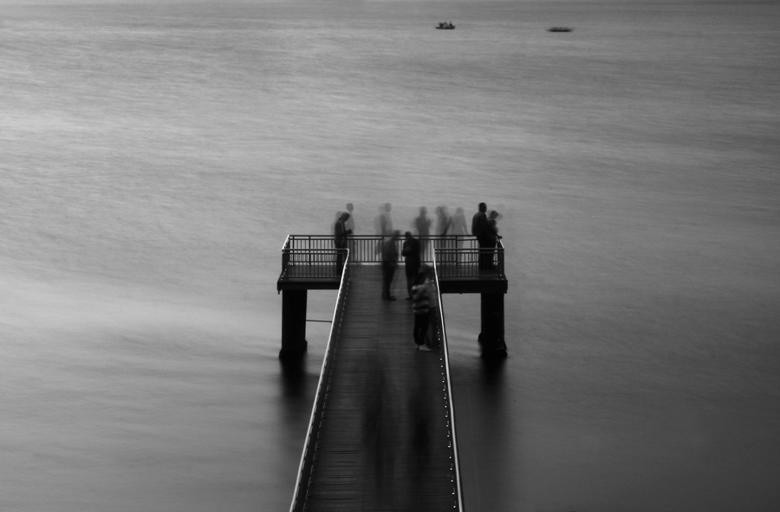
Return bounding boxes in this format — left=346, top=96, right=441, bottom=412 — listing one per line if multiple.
left=382, top=294, right=414, bottom=301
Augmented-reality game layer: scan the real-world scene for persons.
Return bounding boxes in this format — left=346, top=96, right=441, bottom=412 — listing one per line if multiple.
left=381, top=229, right=401, bottom=302
left=422, top=266, right=443, bottom=349
left=409, top=271, right=432, bottom=353
left=333, top=202, right=503, bottom=277
left=401, top=231, right=423, bottom=300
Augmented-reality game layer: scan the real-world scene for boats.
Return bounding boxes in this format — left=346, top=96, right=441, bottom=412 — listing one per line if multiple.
left=435, top=20, right=456, bottom=29
left=546, top=27, right=573, bottom=32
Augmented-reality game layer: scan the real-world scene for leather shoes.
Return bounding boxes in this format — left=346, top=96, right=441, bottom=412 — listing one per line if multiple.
left=416, top=345, right=433, bottom=352
